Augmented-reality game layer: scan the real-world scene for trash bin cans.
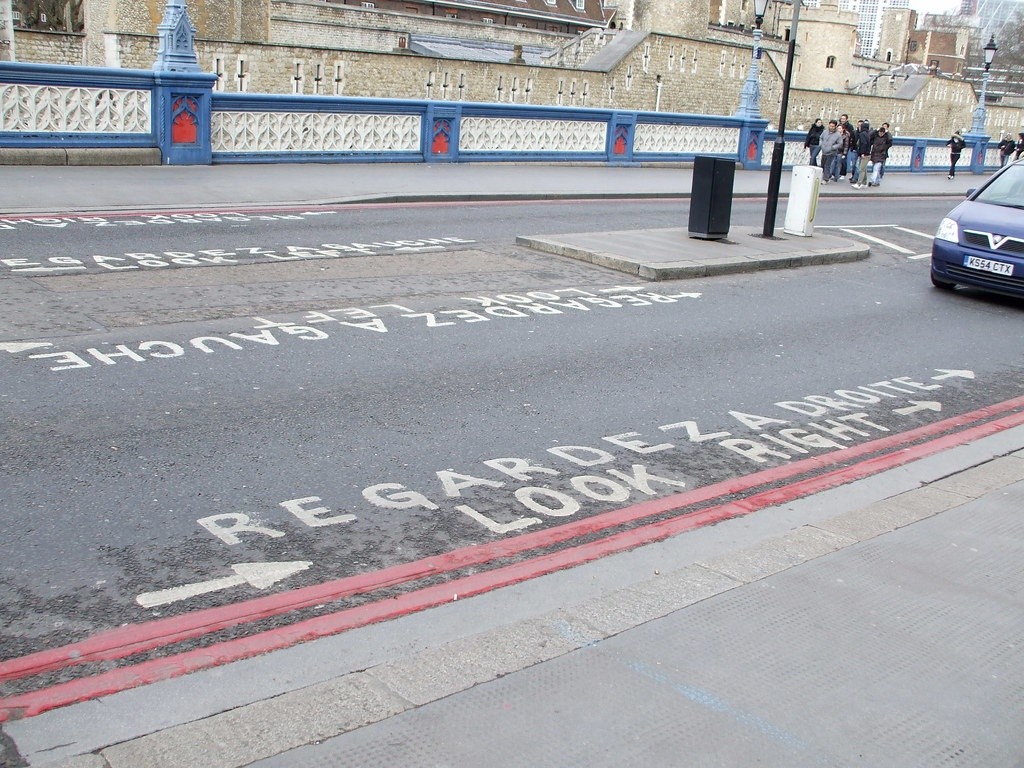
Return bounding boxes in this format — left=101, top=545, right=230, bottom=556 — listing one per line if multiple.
left=784, top=164, right=824, bottom=238
left=687, top=153, right=737, bottom=239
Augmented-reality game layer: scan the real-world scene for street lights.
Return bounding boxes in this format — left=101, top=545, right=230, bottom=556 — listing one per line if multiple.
left=733, top=0, right=769, bottom=120
left=969, top=34, right=998, bottom=136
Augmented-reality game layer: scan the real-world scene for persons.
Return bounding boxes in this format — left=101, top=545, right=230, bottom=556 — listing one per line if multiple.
left=819, top=120, right=843, bottom=185
left=869, top=122, right=893, bottom=187
left=829, top=114, right=875, bottom=183
left=1013, top=133, right=1024, bottom=162
left=998, top=133, right=1015, bottom=168
left=946, top=130, right=966, bottom=180
left=851, top=123, right=871, bottom=190
left=804, top=118, right=825, bottom=165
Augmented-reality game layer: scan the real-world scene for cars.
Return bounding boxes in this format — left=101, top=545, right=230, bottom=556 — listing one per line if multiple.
left=929, top=152, right=1024, bottom=300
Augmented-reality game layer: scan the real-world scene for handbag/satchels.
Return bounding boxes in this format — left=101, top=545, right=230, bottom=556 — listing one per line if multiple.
left=1000, top=151, right=1004, bottom=155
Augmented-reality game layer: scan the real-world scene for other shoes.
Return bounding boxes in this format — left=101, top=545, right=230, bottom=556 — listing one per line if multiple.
left=868, top=181, right=872, bottom=187
left=849, top=177, right=853, bottom=180
left=951, top=176, right=953, bottom=179
left=860, top=184, right=868, bottom=188
left=948, top=176, right=951, bottom=179
left=850, top=180, right=856, bottom=183
left=821, top=180, right=829, bottom=185
left=840, top=176, right=844, bottom=179
left=834, top=178, right=837, bottom=182
left=851, top=184, right=860, bottom=189
left=872, top=183, right=880, bottom=186
